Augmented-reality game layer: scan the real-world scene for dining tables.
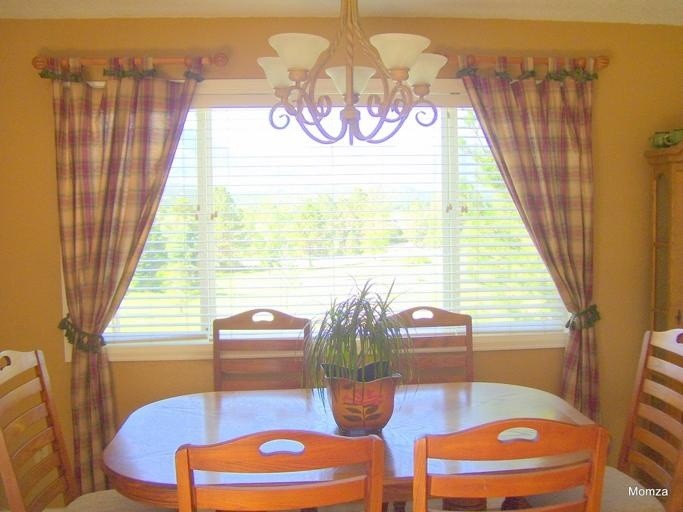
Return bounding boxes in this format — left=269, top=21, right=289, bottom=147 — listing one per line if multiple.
left=298, top=277, right=422, bottom=436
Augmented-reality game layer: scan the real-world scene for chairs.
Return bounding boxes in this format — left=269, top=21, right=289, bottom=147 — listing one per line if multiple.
left=0, top=305, right=682, bottom=512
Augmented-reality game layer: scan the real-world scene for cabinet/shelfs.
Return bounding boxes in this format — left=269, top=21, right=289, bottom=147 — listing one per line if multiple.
left=643, top=141, right=683, bottom=505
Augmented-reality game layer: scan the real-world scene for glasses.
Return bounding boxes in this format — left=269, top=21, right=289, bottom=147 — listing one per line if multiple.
left=255, top=0, right=450, bottom=148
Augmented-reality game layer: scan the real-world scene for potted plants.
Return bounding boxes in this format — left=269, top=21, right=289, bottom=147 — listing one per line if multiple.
left=298, top=277, right=422, bottom=436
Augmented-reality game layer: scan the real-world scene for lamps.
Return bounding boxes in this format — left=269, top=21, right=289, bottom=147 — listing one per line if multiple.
left=255, top=0, right=450, bottom=148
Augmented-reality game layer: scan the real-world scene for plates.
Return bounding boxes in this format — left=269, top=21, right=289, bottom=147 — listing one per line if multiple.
left=643, top=141, right=683, bottom=505
